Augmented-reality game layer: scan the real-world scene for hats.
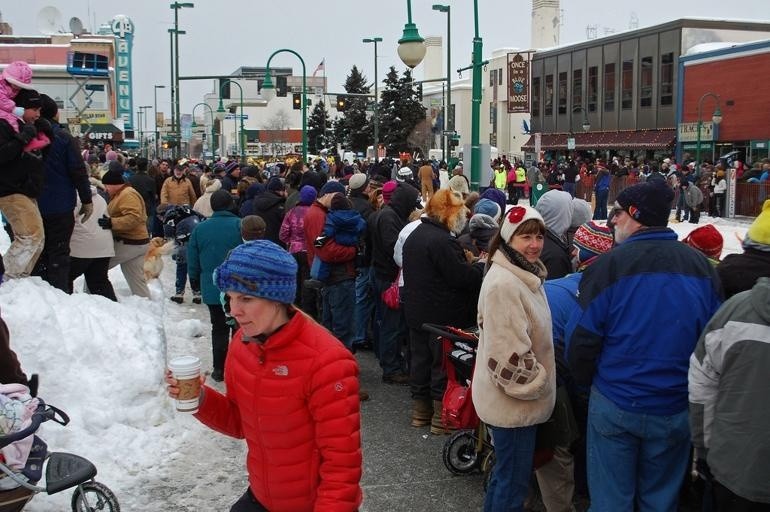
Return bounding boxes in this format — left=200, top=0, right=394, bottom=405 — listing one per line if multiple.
left=468, top=213, right=499, bottom=232
left=663, top=157, right=671, bottom=164
left=39, top=93, right=58, bottom=119
left=298, top=185, right=318, bottom=204
left=569, top=217, right=614, bottom=265
left=741, top=198, right=770, bottom=252
left=3, top=61, right=34, bottom=88
left=88, top=157, right=98, bottom=165
left=209, top=188, right=235, bottom=212
left=395, top=166, right=414, bottom=183
left=223, top=159, right=240, bottom=175
left=499, top=205, right=546, bottom=244
left=330, top=192, right=353, bottom=210
left=319, top=181, right=346, bottom=197
left=98, top=152, right=106, bottom=163
left=480, top=187, right=506, bottom=216
left=681, top=223, right=724, bottom=260
left=212, top=162, right=227, bottom=174
left=474, top=198, right=503, bottom=222
left=348, top=173, right=367, bottom=190
left=247, top=166, right=258, bottom=177
left=716, top=169, right=724, bottom=178
left=106, top=151, right=117, bottom=159
left=415, top=195, right=424, bottom=210
left=446, top=175, right=471, bottom=196
left=211, top=238, right=299, bottom=304
left=382, top=180, right=397, bottom=205
left=613, top=171, right=675, bottom=228
left=101, top=167, right=126, bottom=186
left=239, top=214, right=267, bottom=241
left=266, top=177, right=284, bottom=191
left=14, top=89, right=42, bottom=109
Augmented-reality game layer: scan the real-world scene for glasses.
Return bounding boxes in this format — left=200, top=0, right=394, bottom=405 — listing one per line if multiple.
left=236, top=168, right=241, bottom=171
left=612, top=207, right=625, bottom=216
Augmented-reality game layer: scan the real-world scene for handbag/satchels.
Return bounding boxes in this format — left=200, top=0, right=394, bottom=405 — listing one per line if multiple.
left=382, top=265, right=400, bottom=310
left=442, top=325, right=481, bottom=432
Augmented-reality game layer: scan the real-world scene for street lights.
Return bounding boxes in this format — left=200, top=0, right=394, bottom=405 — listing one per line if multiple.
left=363, top=38, right=383, bottom=162
left=216, top=80, right=248, bottom=170
left=139, top=106, right=153, bottom=158
left=137, top=112, right=144, bottom=141
left=432, top=4, right=451, bottom=165
left=154, top=85, right=166, bottom=158
left=260, top=49, right=307, bottom=168
left=566, top=107, right=590, bottom=159
left=170, top=2, right=194, bottom=159
left=696, top=93, right=722, bottom=177
left=190, top=103, right=214, bottom=169
left=167, top=29, right=186, bottom=160
left=397, top=0, right=489, bottom=191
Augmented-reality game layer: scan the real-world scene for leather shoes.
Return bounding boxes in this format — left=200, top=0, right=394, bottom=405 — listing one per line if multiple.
left=170, top=296, right=184, bottom=304
left=304, top=279, right=323, bottom=290
left=357, top=390, right=367, bottom=402
left=192, top=297, right=201, bottom=304
left=211, top=367, right=225, bottom=382
left=357, top=341, right=372, bottom=352
left=382, top=373, right=411, bottom=386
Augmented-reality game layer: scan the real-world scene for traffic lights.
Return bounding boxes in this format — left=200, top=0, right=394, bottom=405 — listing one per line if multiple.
left=161, top=141, right=178, bottom=149
left=293, top=95, right=301, bottom=109
left=337, top=96, right=345, bottom=111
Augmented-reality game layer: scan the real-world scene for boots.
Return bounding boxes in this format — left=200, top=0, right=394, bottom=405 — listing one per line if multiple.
left=411, top=398, right=434, bottom=427
left=429, top=399, right=458, bottom=436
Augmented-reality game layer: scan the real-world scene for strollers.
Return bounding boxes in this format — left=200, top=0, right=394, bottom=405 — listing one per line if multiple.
left=418, top=322, right=541, bottom=512
left=0, top=396, right=120, bottom=512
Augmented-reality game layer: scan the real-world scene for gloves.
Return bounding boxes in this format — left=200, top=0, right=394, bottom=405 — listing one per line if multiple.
left=78, top=202, right=94, bottom=223
left=688, top=461, right=709, bottom=494
left=313, top=233, right=327, bottom=248
left=35, top=118, right=53, bottom=137
left=97, top=213, right=113, bottom=231
left=20, top=122, right=36, bottom=143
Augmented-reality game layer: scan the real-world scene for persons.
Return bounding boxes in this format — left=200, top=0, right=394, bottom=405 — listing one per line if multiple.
left=527, top=161, right=547, bottom=207
left=85, top=153, right=100, bottom=177
left=35, top=93, right=94, bottom=295
left=187, top=188, right=245, bottom=380
left=193, top=179, right=223, bottom=217
left=158, top=203, right=209, bottom=305
left=304, top=180, right=372, bottom=402
left=418, top=160, right=438, bottom=203
left=85, top=169, right=151, bottom=298
left=214, top=167, right=233, bottom=192
left=284, top=170, right=322, bottom=217
left=279, top=186, right=317, bottom=309
left=164, top=239, right=366, bottom=511
left=287, top=170, right=303, bottom=194
left=536, top=266, right=594, bottom=512
left=439, top=163, right=450, bottom=190
left=252, top=178, right=290, bottom=250
left=547, top=157, right=569, bottom=182
left=123, top=158, right=138, bottom=181
left=513, top=162, right=526, bottom=202
left=0, top=60, right=50, bottom=152
left=303, top=192, right=367, bottom=291
left=731, top=156, right=770, bottom=186
left=347, top=173, right=377, bottom=350
left=200, top=167, right=214, bottom=195
left=571, top=217, right=615, bottom=275
left=611, top=154, right=671, bottom=174
left=474, top=199, right=501, bottom=225
left=532, top=189, right=574, bottom=283
left=682, top=226, right=726, bottom=267
left=161, top=164, right=197, bottom=206
left=469, top=205, right=558, bottom=512
left=672, top=166, right=698, bottom=224
left=593, top=164, right=611, bottom=220
left=382, top=181, right=399, bottom=206
left=493, top=161, right=508, bottom=194
left=348, top=158, right=380, bottom=173
left=68, top=160, right=118, bottom=303
left=155, top=159, right=171, bottom=204
left=505, top=161, right=517, bottom=205
left=463, top=210, right=501, bottom=267
left=679, top=178, right=705, bottom=224
left=718, top=201, right=770, bottom=302
left=712, top=169, right=728, bottom=217
left=127, top=157, right=159, bottom=237
left=239, top=165, right=260, bottom=192
left=239, top=182, right=266, bottom=218
left=686, top=198, right=770, bottom=512
left=567, top=198, right=594, bottom=251
left=185, top=166, right=201, bottom=197
left=381, top=158, right=418, bottom=181
left=101, top=150, right=118, bottom=170
left=268, top=164, right=287, bottom=185
left=569, top=155, right=593, bottom=174
left=404, top=189, right=490, bottom=434
left=101, top=160, right=126, bottom=185
left=1, top=88, right=60, bottom=283
left=568, top=175, right=728, bottom=512
left=371, top=182, right=426, bottom=388
left=322, top=154, right=347, bottom=180
left=222, top=161, right=240, bottom=192
